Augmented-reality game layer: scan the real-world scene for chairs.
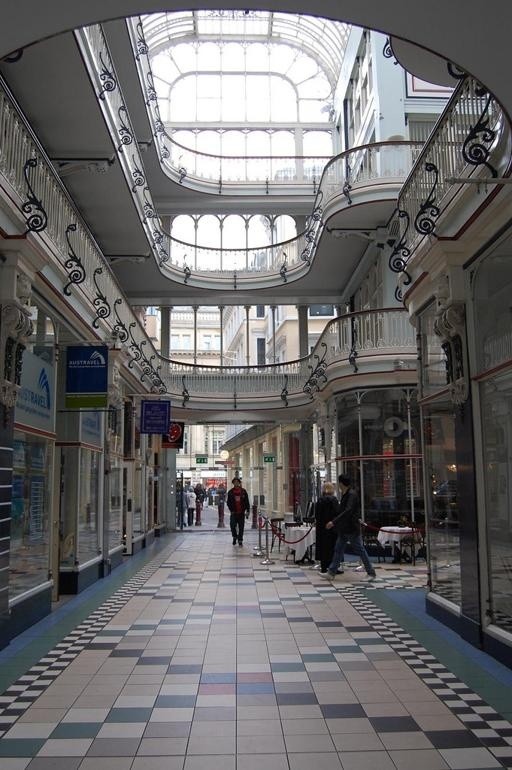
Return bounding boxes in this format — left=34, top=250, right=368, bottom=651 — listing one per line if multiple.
left=414, top=521, right=428, bottom=564
left=360, top=520, right=386, bottom=563
left=269, top=516, right=315, bottom=567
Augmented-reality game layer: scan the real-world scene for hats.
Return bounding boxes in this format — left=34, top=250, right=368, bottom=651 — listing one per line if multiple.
left=232, top=477, right=241, bottom=483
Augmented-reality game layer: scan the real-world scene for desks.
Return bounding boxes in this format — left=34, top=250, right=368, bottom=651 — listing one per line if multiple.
left=377, top=526, right=421, bottom=564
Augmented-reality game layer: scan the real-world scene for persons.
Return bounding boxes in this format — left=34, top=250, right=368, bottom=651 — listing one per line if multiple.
left=318, top=474, right=376, bottom=582
left=227, top=478, right=250, bottom=546
left=315, top=482, right=339, bottom=575
left=183, top=481, right=226, bottom=526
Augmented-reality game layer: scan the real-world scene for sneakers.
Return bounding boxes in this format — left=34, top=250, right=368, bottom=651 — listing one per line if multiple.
left=318, top=572, right=334, bottom=580
left=360, top=575, right=375, bottom=581
